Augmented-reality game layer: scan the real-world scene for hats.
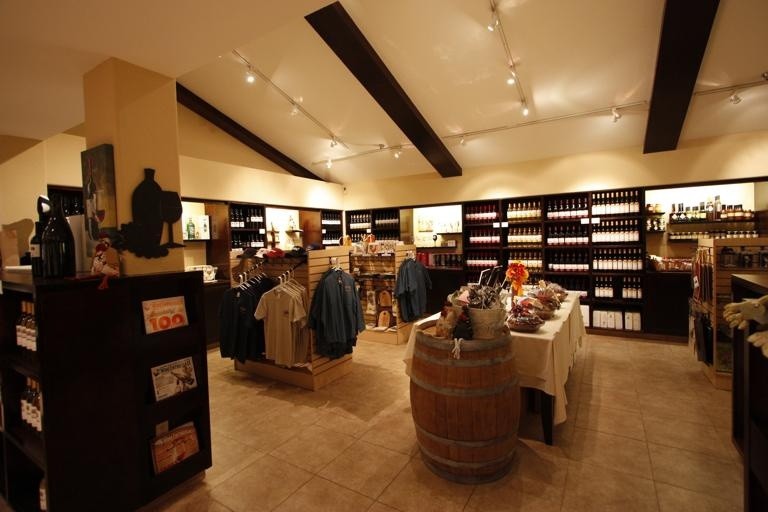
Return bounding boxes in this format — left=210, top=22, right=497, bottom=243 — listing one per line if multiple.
left=236, top=242, right=323, bottom=259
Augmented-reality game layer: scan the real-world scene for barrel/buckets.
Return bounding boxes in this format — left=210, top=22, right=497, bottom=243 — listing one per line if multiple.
left=407, top=319, right=522, bottom=484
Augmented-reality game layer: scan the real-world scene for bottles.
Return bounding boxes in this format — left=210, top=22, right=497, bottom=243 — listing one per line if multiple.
left=39, top=474, right=48, bottom=511
left=462, top=189, right=642, bottom=300
left=646, top=194, right=758, bottom=242
left=350, top=212, right=371, bottom=242
left=416, top=252, right=462, bottom=269
left=15, top=299, right=39, bottom=375
left=321, top=211, right=343, bottom=247
left=231, top=206, right=266, bottom=250
left=650, top=255, right=692, bottom=272
left=29, top=221, right=43, bottom=278
left=39, top=192, right=77, bottom=278
left=186, top=218, right=196, bottom=240
left=19, top=377, right=43, bottom=444
left=374, top=209, right=400, bottom=240
left=718, top=244, right=768, bottom=268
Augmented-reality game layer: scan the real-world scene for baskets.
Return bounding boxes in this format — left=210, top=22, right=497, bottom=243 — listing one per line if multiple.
left=464, top=305, right=506, bottom=339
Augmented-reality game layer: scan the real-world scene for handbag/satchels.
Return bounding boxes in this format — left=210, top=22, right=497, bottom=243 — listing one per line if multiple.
left=688, top=248, right=714, bottom=367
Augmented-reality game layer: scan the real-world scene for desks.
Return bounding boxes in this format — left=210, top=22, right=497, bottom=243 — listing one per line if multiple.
left=407, top=282, right=582, bottom=448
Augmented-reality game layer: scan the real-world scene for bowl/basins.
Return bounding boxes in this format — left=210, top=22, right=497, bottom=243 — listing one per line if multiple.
left=506, top=289, right=569, bottom=333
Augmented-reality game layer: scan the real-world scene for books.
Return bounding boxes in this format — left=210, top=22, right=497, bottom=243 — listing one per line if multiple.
left=148, top=420, right=201, bottom=478
left=140, top=295, right=189, bottom=335
left=150, top=356, right=198, bottom=406
